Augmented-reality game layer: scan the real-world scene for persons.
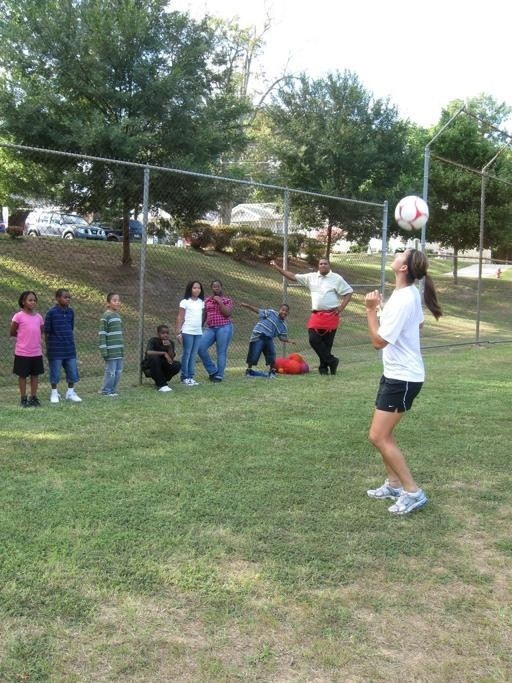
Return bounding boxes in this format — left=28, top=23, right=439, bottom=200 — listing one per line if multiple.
left=44, top=288, right=84, bottom=401
left=174, top=280, right=205, bottom=386
left=268, top=257, right=353, bottom=375
left=97, top=290, right=125, bottom=395
left=141, top=324, right=182, bottom=391
left=363, top=249, right=441, bottom=514
left=8, top=289, right=47, bottom=405
left=198, top=279, right=234, bottom=382
left=240, top=300, right=296, bottom=376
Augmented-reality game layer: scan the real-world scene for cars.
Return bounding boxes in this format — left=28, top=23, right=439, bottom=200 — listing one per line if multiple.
left=0, top=198, right=148, bottom=240
left=324, top=241, right=454, bottom=259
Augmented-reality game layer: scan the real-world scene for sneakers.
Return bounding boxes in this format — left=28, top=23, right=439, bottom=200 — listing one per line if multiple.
left=20, top=390, right=83, bottom=410
left=387, top=487, right=428, bottom=516
left=246, top=368, right=277, bottom=378
left=318, top=358, right=340, bottom=376
left=97, top=370, right=224, bottom=397
left=366, top=479, right=404, bottom=500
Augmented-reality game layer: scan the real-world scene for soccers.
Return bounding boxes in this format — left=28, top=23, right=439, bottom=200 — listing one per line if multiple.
left=393, top=195, right=429, bottom=231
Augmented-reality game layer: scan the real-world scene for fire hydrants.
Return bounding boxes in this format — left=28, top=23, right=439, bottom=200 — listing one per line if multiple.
left=496, top=267, right=502, bottom=278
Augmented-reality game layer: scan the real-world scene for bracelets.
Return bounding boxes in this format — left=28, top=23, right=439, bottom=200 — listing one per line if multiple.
left=176, top=332, right=180, bottom=336
